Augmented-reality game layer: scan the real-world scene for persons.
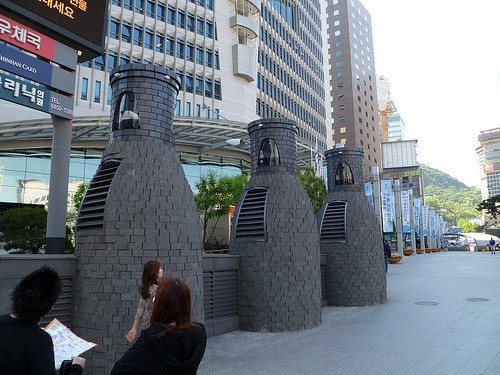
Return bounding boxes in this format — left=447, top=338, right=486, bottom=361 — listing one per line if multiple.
left=383, top=238, right=391, bottom=272
left=490, top=238, right=495, bottom=254
left=110, top=278, right=207, bottom=375
left=0, top=266, right=86, bottom=375
left=126, top=261, right=163, bottom=342
